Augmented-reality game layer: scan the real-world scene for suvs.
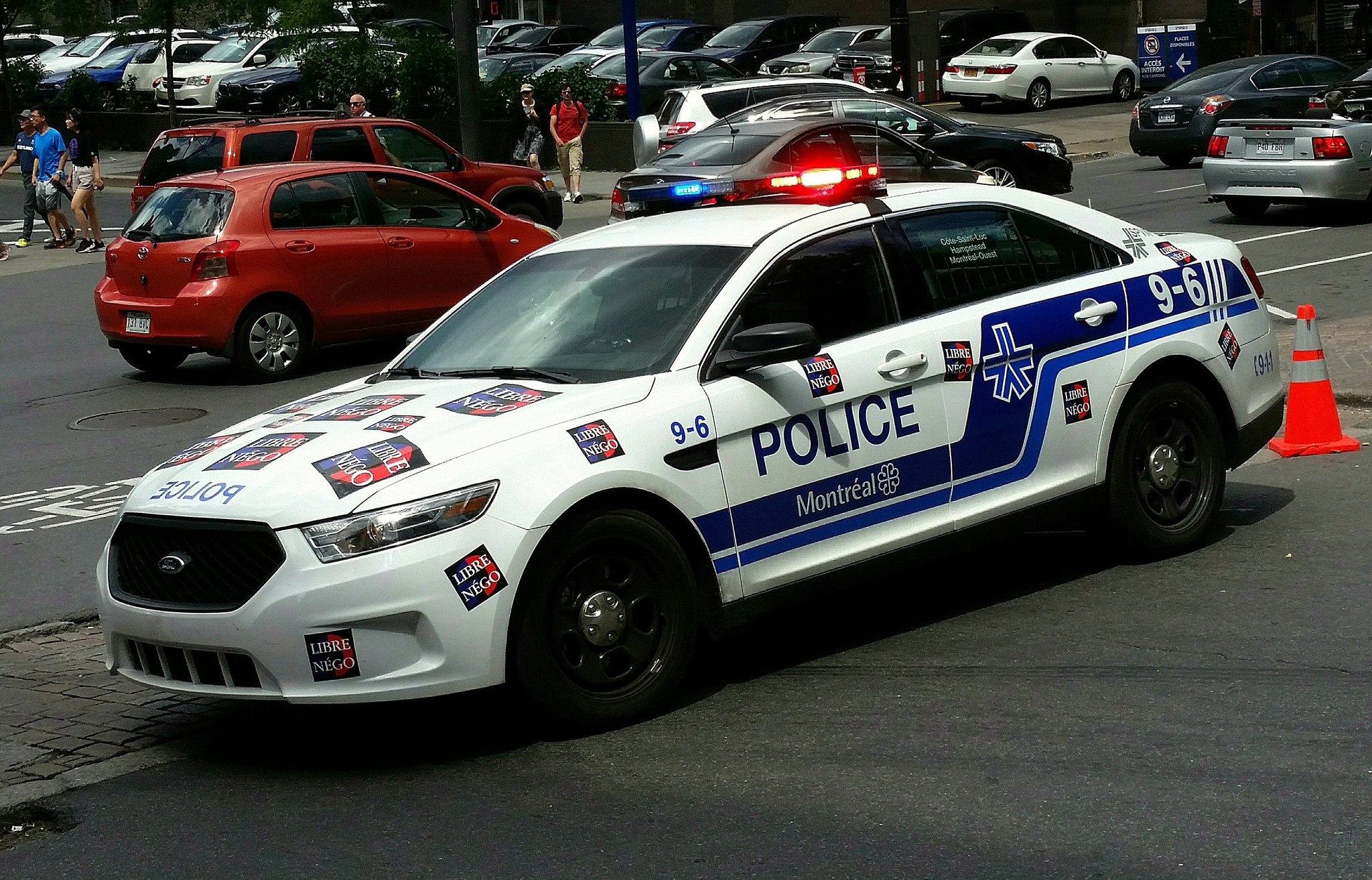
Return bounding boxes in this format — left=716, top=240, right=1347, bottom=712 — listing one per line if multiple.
left=830, top=6, right=1040, bottom=94
left=132, top=108, right=564, bottom=237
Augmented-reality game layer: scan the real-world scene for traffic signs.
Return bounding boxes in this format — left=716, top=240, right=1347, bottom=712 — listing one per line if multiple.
left=1165, top=23, right=1198, bottom=84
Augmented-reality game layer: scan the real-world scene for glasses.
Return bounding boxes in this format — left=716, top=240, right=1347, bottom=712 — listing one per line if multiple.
left=29, top=115, right=42, bottom=118
left=72, top=108, right=78, bottom=113
left=349, top=102, right=363, bottom=107
left=562, top=91, right=571, bottom=94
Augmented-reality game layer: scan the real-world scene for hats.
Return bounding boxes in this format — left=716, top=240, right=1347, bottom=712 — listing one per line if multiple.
left=521, top=84, right=534, bottom=93
left=17, top=110, right=31, bottom=119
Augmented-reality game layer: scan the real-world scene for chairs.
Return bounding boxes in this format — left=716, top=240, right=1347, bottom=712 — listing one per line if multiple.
left=676, top=65, right=689, bottom=75
left=989, top=47, right=998, bottom=53
left=809, top=141, right=831, bottom=151
left=491, top=30, right=500, bottom=38
left=1305, top=108, right=1332, bottom=118
left=1001, top=50, right=1012, bottom=54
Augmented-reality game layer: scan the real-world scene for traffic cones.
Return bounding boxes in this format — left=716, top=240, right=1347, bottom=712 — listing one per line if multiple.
left=1274, top=299, right=1362, bottom=459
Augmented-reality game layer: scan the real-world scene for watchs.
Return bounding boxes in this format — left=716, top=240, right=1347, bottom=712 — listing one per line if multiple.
left=55, top=170, right=63, bottom=175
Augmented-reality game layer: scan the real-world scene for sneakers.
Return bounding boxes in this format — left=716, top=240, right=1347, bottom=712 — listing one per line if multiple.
left=75, top=237, right=95, bottom=252
left=15, top=238, right=33, bottom=247
left=65, top=225, right=77, bottom=246
left=564, top=191, right=572, bottom=202
left=43, top=239, right=66, bottom=248
left=44, top=230, right=66, bottom=242
left=571, top=192, right=583, bottom=203
left=87, top=239, right=106, bottom=252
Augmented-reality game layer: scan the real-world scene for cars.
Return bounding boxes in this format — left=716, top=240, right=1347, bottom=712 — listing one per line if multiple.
left=940, top=31, right=1141, bottom=110
left=1127, top=52, right=1371, bottom=218
left=94, top=159, right=565, bottom=384
left=606, top=73, right=1074, bottom=225
left=96, top=167, right=1294, bottom=741
left=2, top=14, right=889, bottom=123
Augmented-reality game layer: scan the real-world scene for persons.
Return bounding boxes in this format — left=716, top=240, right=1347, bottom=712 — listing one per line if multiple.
left=0, top=110, right=55, bottom=247
left=0, top=237, right=11, bottom=261
left=550, top=85, right=590, bottom=204
left=350, top=95, right=388, bottom=151
left=65, top=108, right=107, bottom=253
left=1324, top=90, right=1352, bottom=120
left=30, top=104, right=77, bottom=249
left=513, top=84, right=549, bottom=171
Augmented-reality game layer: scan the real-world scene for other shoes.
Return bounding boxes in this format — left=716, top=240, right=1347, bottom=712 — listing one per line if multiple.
left=0, top=245, right=11, bottom=261
